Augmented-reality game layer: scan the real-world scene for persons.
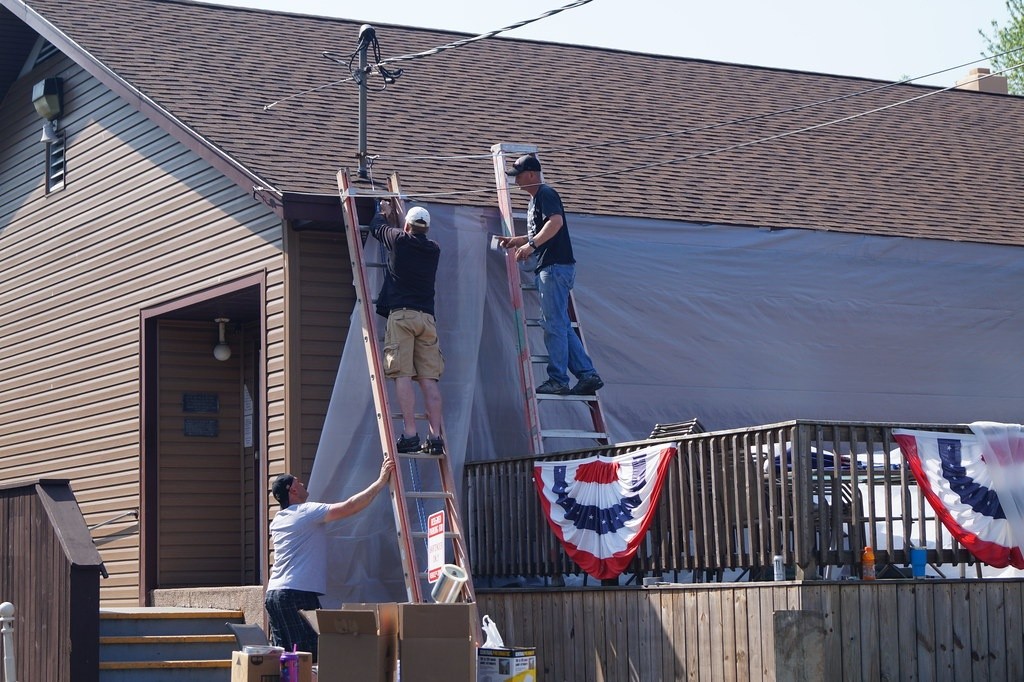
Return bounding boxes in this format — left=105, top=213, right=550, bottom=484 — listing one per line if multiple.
left=265, top=458, right=396, bottom=663
left=371, top=200, right=445, bottom=456
left=494, top=155, right=605, bottom=395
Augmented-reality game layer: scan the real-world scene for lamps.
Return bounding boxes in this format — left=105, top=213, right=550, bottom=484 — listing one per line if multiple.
left=31, top=77, right=59, bottom=120
left=430, top=563, right=469, bottom=604
left=214, top=317, right=232, bottom=360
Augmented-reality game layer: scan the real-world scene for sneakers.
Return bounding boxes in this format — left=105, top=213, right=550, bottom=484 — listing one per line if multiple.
left=570, top=374, right=604, bottom=394
left=421, top=435, right=443, bottom=455
left=397, top=432, right=421, bottom=453
left=536, top=378, right=570, bottom=395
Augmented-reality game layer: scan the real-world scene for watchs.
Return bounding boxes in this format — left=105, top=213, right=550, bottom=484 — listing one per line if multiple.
left=528, top=239, right=538, bottom=251
left=379, top=212, right=387, bottom=218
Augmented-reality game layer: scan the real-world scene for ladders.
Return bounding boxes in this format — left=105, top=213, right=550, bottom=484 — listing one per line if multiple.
left=491, top=142, right=646, bottom=589
left=336, top=167, right=484, bottom=647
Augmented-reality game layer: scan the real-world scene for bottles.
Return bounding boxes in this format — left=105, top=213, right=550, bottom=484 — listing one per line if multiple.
left=773, top=553, right=783, bottom=581
left=862, top=546, right=876, bottom=581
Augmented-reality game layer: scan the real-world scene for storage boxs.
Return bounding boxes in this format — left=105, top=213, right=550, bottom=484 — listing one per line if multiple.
left=398, top=601, right=478, bottom=682
left=231, top=650, right=313, bottom=682
left=318, top=601, right=399, bottom=682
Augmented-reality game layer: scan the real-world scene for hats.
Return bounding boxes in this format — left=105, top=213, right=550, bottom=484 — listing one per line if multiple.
left=272, top=474, right=294, bottom=511
left=404, top=207, right=430, bottom=227
left=505, top=155, right=541, bottom=176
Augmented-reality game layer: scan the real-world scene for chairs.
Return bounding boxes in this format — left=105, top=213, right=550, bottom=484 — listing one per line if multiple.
left=225, top=621, right=268, bottom=651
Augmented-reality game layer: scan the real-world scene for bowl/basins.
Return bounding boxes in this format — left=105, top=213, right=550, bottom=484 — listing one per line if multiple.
left=242, top=645, right=284, bottom=658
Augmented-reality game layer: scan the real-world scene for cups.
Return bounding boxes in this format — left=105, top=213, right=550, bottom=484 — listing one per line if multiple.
left=912, top=547, right=927, bottom=580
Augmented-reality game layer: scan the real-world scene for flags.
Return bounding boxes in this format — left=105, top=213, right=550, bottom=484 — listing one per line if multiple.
left=533, top=442, right=678, bottom=580
left=892, top=429, right=1024, bottom=570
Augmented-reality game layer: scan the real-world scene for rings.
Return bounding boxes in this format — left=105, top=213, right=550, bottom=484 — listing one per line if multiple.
left=521, top=256, right=525, bottom=258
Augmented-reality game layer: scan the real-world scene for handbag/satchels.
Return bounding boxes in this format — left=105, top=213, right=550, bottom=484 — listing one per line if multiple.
left=481, top=615, right=504, bottom=648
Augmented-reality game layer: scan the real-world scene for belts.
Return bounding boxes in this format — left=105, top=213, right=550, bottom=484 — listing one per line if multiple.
left=386, top=307, right=435, bottom=315
